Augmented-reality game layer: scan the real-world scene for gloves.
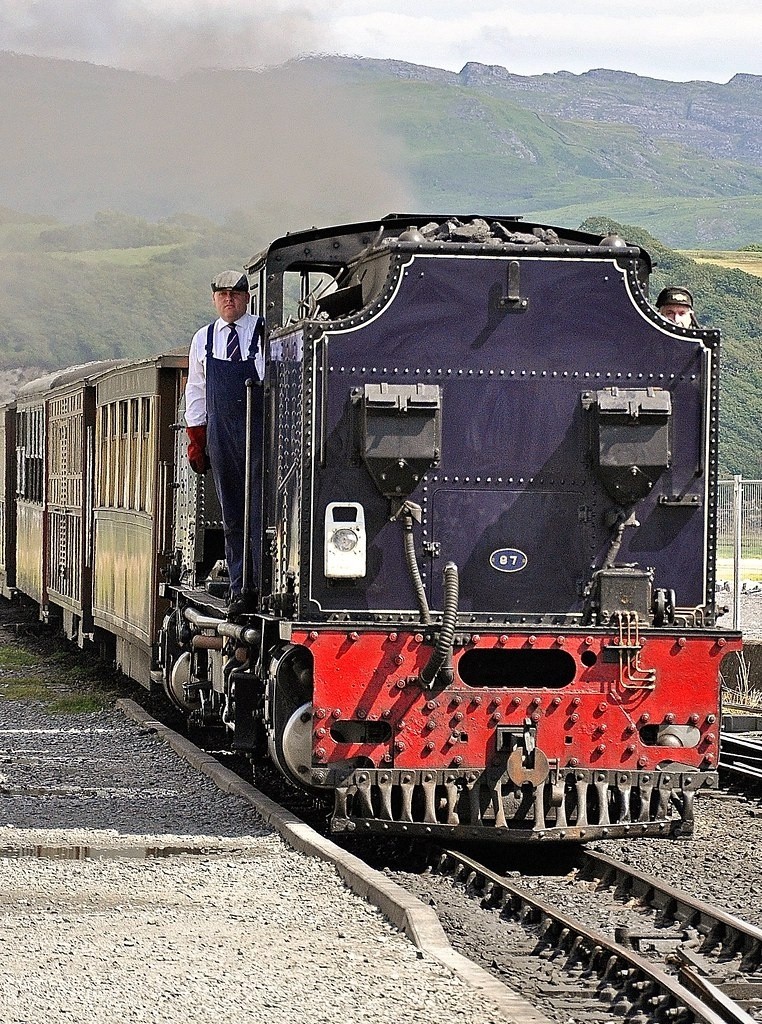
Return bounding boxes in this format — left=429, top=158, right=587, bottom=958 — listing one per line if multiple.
left=186, top=425, right=211, bottom=474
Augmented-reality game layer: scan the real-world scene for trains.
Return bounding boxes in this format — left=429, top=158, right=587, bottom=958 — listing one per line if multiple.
left=0, top=214, right=739, bottom=842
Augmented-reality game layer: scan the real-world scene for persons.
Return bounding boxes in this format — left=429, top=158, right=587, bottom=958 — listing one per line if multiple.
left=184, top=269, right=265, bottom=615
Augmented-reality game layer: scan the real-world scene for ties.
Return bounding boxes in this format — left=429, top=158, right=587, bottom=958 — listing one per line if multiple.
left=226, top=322, right=242, bottom=362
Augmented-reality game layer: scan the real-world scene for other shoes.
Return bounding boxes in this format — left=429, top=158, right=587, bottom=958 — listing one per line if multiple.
left=226, top=593, right=259, bottom=615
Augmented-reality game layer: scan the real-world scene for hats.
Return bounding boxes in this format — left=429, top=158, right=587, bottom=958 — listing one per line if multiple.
left=211, top=270, right=248, bottom=293
